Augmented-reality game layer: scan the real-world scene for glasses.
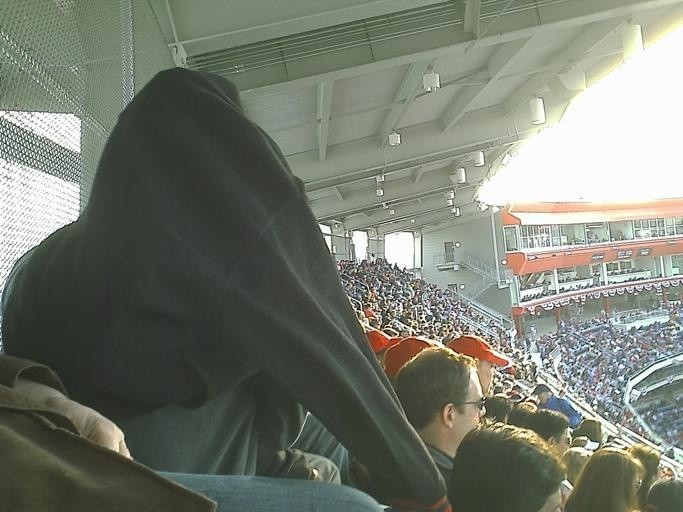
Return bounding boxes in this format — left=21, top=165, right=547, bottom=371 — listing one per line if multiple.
left=454, top=395, right=486, bottom=409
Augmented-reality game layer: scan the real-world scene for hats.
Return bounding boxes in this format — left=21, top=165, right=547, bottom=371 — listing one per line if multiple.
left=570, top=435, right=626, bottom=450
left=647, top=478, right=683, bottom=512
left=366, top=329, right=508, bottom=376
left=531, top=384, right=551, bottom=396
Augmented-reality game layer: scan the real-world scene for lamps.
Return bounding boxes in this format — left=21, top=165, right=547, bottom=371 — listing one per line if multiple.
left=621, top=18, right=644, bottom=65
left=529, top=94, right=546, bottom=125
left=376, top=173, right=396, bottom=215
left=387, top=131, right=401, bottom=147
left=446, top=189, right=460, bottom=217
left=452, top=151, right=484, bottom=184
left=421, top=65, right=441, bottom=92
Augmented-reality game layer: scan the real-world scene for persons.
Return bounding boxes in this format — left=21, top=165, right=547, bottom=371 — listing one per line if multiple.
left=0, top=227, right=683, bottom=512
left=1, top=67, right=452, bottom=511
